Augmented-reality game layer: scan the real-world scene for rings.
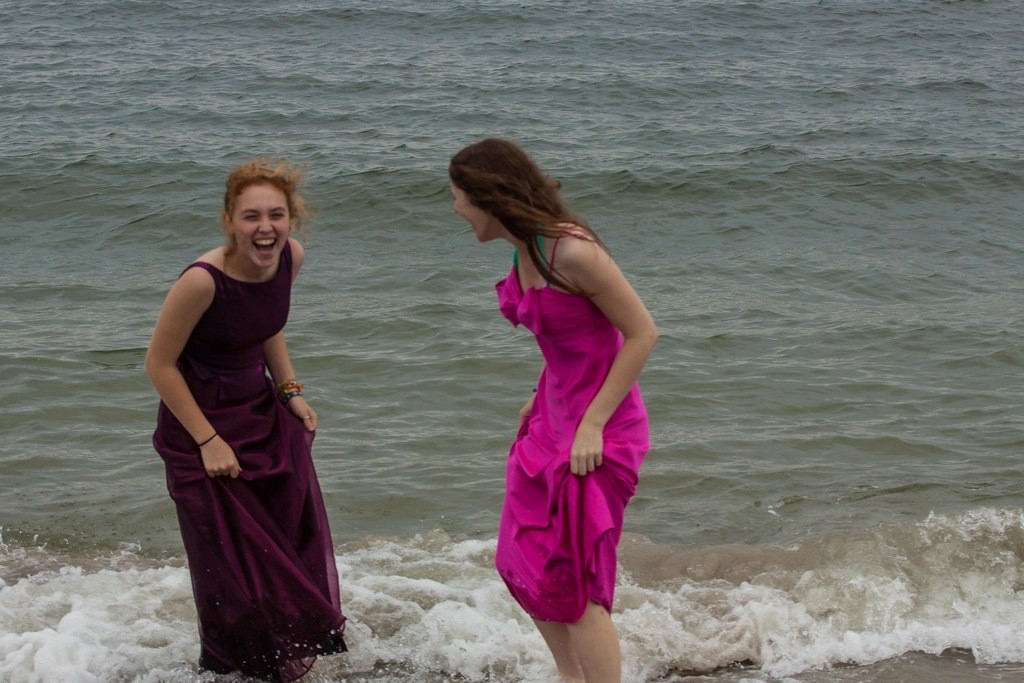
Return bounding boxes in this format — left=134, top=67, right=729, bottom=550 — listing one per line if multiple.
left=303, top=416, right=311, bottom=419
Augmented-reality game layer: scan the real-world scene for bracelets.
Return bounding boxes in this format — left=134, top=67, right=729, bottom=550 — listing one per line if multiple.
left=276, top=378, right=302, bottom=405
left=533, top=389, right=537, bottom=392
left=199, top=433, right=218, bottom=447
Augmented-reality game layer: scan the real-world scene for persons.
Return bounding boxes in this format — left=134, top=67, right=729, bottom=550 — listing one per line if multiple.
left=145, top=161, right=349, bottom=683
left=448, top=139, right=658, bottom=683
